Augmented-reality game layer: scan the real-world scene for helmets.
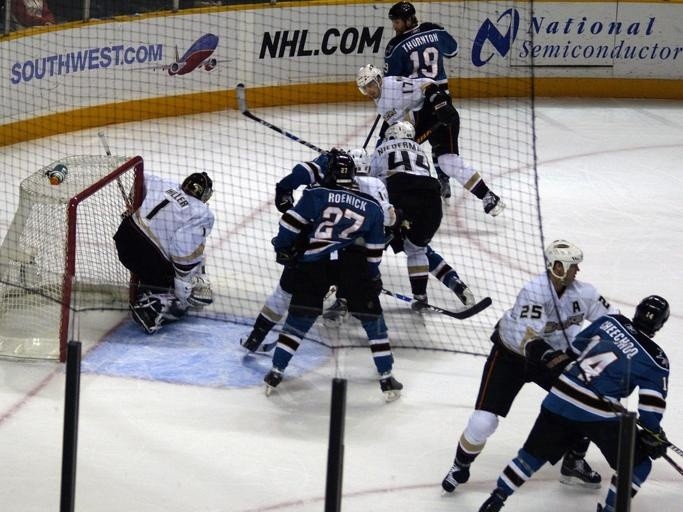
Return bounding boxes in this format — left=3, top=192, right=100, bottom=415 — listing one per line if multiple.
left=388, top=2, right=417, bottom=21
left=355, top=63, right=383, bottom=96
left=181, top=170, right=215, bottom=203
left=326, top=153, right=357, bottom=184
left=346, top=147, right=372, bottom=174
left=541, top=238, right=584, bottom=281
left=633, top=295, right=671, bottom=338
left=384, top=120, right=417, bottom=142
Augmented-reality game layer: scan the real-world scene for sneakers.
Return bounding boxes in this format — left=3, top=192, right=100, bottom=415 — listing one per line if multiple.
left=378, top=374, right=404, bottom=393
left=134, top=298, right=157, bottom=334
left=481, top=189, right=500, bottom=214
left=244, top=328, right=265, bottom=354
left=448, top=275, right=469, bottom=306
left=413, top=294, right=428, bottom=304
left=560, top=454, right=602, bottom=484
left=478, top=493, right=504, bottom=512
left=438, top=176, right=451, bottom=200
left=321, top=299, right=350, bottom=320
left=441, top=462, right=468, bottom=492
left=262, top=368, right=283, bottom=390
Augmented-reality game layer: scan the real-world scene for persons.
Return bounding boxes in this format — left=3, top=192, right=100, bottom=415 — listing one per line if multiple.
left=264, top=156, right=405, bottom=393
left=356, top=64, right=498, bottom=214
left=476, top=293, right=669, bottom=510
left=113, top=172, right=213, bottom=335
left=382, top=1, right=460, bottom=199
left=10, top=0, right=54, bottom=29
left=440, top=239, right=619, bottom=495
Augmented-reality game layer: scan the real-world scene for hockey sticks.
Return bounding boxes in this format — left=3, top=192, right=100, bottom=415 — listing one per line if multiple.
left=380, top=286, right=493, bottom=320
left=237, top=83, right=329, bottom=154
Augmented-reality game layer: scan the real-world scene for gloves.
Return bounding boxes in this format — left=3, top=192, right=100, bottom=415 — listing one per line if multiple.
left=640, top=427, right=669, bottom=459
left=275, top=182, right=295, bottom=213
left=432, top=93, right=456, bottom=126
left=275, top=244, right=300, bottom=266
left=542, top=348, right=572, bottom=381
